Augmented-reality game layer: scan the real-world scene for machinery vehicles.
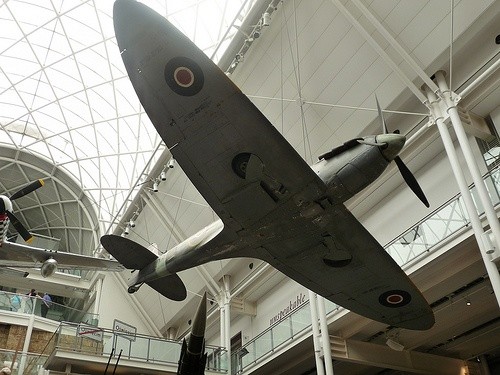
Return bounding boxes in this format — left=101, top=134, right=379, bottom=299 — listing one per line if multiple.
left=176, top=292, right=208, bottom=375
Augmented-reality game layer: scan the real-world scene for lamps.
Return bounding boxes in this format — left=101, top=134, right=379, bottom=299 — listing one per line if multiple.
left=236, top=54, right=244, bottom=62
left=161, top=172, right=166, bottom=181
left=125, top=227, right=130, bottom=235
left=129, top=221, right=136, bottom=228
left=169, top=160, right=175, bottom=168
left=264, top=13, right=270, bottom=27
left=153, top=183, right=159, bottom=193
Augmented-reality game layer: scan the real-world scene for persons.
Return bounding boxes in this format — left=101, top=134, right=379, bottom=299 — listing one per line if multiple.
left=8, top=289, right=22, bottom=312
left=41, top=292, right=51, bottom=318
left=25, top=289, right=36, bottom=314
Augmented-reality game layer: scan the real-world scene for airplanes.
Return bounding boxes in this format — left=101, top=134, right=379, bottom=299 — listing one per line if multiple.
left=99, top=0, right=436, bottom=331
left=0, top=179, right=126, bottom=278
